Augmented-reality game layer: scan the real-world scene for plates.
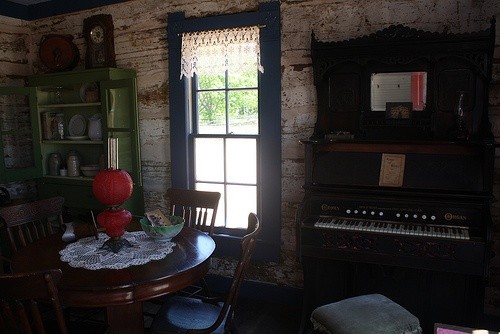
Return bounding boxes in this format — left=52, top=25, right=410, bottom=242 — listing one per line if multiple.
left=79, top=80, right=99, bottom=103
left=66, top=135, right=88, bottom=140
left=68, top=113, right=86, bottom=136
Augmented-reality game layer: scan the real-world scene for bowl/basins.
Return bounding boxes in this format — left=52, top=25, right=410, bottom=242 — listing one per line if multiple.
left=140, top=215, right=186, bottom=242
left=81, top=165, right=100, bottom=177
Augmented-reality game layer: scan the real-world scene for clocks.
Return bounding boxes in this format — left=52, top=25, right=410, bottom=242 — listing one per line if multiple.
left=83, top=12, right=117, bottom=68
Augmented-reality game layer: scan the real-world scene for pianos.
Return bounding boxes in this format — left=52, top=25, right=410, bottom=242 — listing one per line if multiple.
left=295, top=135, right=496, bottom=334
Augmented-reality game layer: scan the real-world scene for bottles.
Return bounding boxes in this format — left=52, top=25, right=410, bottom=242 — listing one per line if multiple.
left=48, top=152, right=61, bottom=175
left=99, top=152, right=106, bottom=170
left=67, top=151, right=81, bottom=177
left=85, top=82, right=97, bottom=103
left=56, top=113, right=65, bottom=140
left=88, top=113, right=103, bottom=140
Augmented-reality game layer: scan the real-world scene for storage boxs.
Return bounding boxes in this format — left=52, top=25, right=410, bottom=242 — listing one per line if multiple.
left=42, top=111, right=64, bottom=139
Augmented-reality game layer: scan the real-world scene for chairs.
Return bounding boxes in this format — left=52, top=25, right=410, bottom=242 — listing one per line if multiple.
left=166, top=188, right=221, bottom=298
left=0, top=196, right=64, bottom=253
left=0, top=270, right=111, bottom=334
left=151, top=213, right=262, bottom=334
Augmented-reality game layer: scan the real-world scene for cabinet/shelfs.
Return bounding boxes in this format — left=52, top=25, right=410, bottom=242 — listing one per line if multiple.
left=0, top=69, right=144, bottom=224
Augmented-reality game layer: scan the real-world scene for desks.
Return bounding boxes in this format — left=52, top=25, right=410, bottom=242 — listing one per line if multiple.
left=22, top=215, right=217, bottom=334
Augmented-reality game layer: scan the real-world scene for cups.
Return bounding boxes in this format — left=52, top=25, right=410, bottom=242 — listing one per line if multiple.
left=59, top=169, right=67, bottom=175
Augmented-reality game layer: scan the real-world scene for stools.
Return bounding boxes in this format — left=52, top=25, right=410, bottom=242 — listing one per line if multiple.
left=309, top=293, right=423, bottom=334
